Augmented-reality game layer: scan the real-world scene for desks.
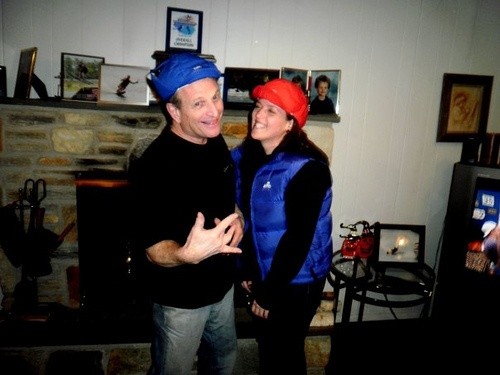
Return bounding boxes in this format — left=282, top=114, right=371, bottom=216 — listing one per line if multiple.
left=328, top=250, right=436, bottom=323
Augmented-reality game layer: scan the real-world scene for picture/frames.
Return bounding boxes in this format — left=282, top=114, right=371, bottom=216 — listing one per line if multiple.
left=61, top=52, right=105, bottom=105
left=166, top=7, right=203, bottom=54
left=98, top=64, right=149, bottom=108
left=223, top=67, right=280, bottom=111
left=436, top=73, right=494, bottom=142
left=13, top=47, right=38, bottom=98
left=281, top=67, right=342, bottom=116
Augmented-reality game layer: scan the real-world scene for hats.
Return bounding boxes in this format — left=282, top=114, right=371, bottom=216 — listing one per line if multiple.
left=149, top=51, right=221, bottom=103
left=252, top=78, right=310, bottom=136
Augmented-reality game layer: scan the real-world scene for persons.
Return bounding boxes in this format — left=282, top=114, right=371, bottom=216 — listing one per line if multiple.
left=480, top=225, right=500, bottom=278
left=309, top=75, right=335, bottom=114
left=117, top=75, right=138, bottom=94
left=230, top=79, right=332, bottom=375
left=129, top=52, right=243, bottom=375
left=78, top=62, right=88, bottom=82
